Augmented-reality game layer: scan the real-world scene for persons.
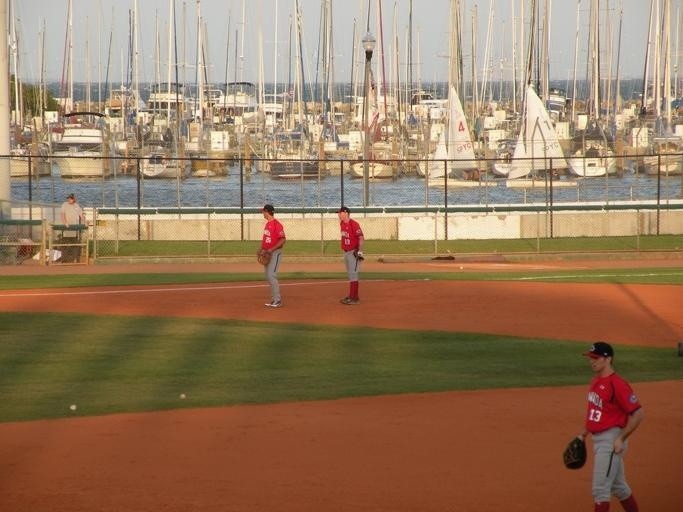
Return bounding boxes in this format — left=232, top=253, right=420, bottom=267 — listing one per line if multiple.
left=336, top=206, right=364, bottom=306
left=561, top=340, right=646, bottom=511
left=257, top=204, right=286, bottom=309
left=59, top=193, right=90, bottom=240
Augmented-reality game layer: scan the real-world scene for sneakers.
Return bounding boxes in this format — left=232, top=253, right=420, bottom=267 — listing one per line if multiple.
left=340, top=297, right=360, bottom=304
left=264, top=300, right=282, bottom=308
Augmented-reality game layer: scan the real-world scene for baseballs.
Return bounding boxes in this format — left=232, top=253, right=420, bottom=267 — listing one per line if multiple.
left=461, top=267, right=464, bottom=270
left=70, top=405, right=76, bottom=410
left=180, top=393, right=186, bottom=399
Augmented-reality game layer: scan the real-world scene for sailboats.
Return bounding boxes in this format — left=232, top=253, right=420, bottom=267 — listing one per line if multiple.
left=10, top=0, right=683, bottom=192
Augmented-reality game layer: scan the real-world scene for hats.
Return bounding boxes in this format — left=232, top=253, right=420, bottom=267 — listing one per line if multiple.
left=582, top=342, right=613, bottom=358
left=259, top=205, right=275, bottom=213
left=336, top=207, right=350, bottom=213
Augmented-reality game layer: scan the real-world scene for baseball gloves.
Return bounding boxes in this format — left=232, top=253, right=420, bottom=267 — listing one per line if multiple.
left=257, top=248, right=271, bottom=266
left=562, top=437, right=587, bottom=469
